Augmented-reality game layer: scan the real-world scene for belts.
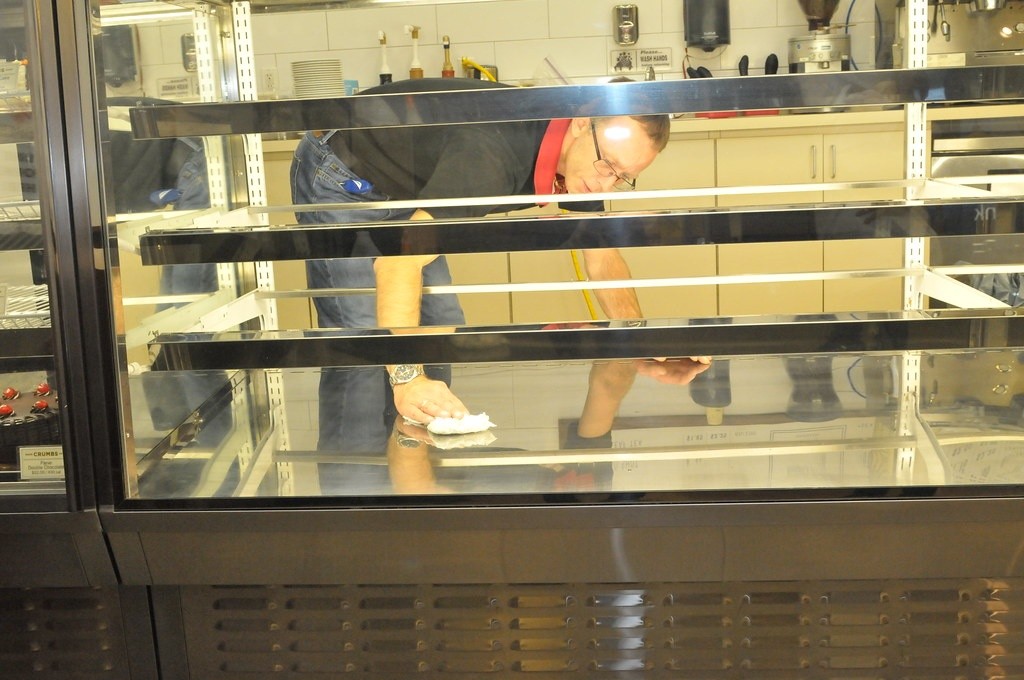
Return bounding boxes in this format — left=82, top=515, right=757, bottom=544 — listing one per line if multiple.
left=158, top=136, right=203, bottom=191
left=322, top=130, right=358, bottom=170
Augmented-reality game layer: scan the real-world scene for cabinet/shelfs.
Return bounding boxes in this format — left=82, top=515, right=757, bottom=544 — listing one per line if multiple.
left=262, top=119, right=933, bottom=329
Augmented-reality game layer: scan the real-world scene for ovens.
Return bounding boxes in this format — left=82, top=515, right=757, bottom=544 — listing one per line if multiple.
left=930, top=140, right=1024, bottom=311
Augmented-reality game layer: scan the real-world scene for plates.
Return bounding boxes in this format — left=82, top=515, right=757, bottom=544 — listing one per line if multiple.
left=290, top=59, right=346, bottom=99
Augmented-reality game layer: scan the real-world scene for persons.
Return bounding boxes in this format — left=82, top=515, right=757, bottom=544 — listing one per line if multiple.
left=387, top=361, right=639, bottom=493
left=17, top=97, right=233, bottom=450
left=290, top=78, right=713, bottom=455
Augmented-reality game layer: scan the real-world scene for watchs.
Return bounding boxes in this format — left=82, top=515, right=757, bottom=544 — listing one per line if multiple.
left=389, top=364, right=426, bottom=389
left=393, top=422, right=425, bottom=448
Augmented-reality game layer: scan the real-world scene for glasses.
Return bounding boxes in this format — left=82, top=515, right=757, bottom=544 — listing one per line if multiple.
left=590, top=118, right=635, bottom=192
